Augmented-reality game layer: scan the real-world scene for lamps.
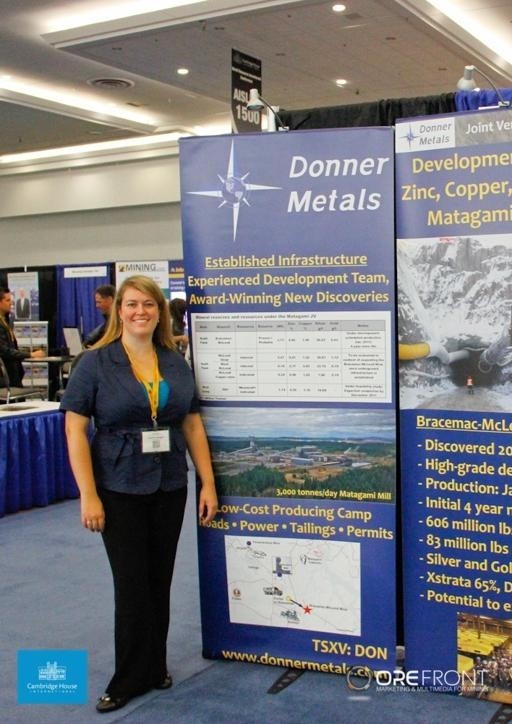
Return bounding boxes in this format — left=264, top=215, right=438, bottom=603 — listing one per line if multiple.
left=243, top=85, right=287, bottom=133
left=456, top=62, right=511, bottom=109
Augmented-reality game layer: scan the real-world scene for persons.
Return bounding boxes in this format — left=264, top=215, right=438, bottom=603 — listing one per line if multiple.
left=81, top=284, right=116, bottom=351
left=58, top=274, right=219, bottom=711
left=16, top=289, right=30, bottom=318
left=474, top=648, right=512, bottom=691
left=0, top=289, right=46, bottom=408
left=173, top=333, right=188, bottom=345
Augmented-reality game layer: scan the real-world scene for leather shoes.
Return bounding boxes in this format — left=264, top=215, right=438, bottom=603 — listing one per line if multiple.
left=96, top=694, right=129, bottom=712
left=153, top=672, right=171, bottom=690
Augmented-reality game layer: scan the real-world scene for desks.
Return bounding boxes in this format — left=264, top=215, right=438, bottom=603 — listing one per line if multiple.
left=0, top=398, right=93, bottom=519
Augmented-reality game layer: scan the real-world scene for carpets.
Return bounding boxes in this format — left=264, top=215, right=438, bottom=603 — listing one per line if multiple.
left=106, top=658, right=510, bottom=722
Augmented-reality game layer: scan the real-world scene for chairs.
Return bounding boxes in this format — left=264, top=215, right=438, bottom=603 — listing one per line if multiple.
left=0, top=357, right=47, bottom=403
